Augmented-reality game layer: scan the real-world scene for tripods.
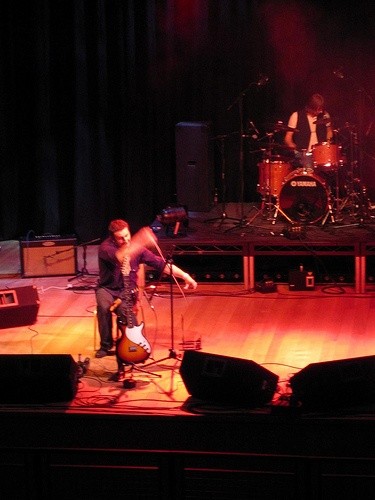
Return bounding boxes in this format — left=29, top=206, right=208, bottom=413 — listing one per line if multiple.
left=210, top=81, right=375, bottom=235
left=138, top=244, right=183, bottom=369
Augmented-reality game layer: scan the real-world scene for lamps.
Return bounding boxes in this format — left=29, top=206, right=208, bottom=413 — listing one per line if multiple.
left=160, top=206, right=187, bottom=238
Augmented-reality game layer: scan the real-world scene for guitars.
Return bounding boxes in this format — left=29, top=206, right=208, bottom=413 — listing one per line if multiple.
left=113, top=255, right=152, bottom=364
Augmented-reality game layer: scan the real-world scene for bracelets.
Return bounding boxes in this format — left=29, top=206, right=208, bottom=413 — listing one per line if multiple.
left=327, top=137, right=332, bottom=141
left=182, top=273, right=189, bottom=280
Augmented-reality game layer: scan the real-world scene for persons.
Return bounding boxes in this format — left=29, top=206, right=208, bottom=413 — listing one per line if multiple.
left=96, top=220, right=197, bottom=358
left=284, top=93, right=333, bottom=211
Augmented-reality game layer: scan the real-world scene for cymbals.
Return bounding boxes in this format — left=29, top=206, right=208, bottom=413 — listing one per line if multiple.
left=268, top=122, right=299, bottom=133
left=267, top=141, right=298, bottom=151
left=313, top=116, right=336, bottom=125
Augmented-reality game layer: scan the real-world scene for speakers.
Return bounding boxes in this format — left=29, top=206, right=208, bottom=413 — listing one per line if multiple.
left=75, top=245, right=101, bottom=274
left=175, top=121, right=215, bottom=212
left=180, top=349, right=280, bottom=407
left=0, top=353, right=79, bottom=405
left=0, top=286, right=41, bottom=328
left=21, top=239, right=78, bottom=277
left=290, top=355, right=375, bottom=412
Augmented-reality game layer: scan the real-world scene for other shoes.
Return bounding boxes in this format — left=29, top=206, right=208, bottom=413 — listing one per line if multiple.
left=95, top=345, right=112, bottom=358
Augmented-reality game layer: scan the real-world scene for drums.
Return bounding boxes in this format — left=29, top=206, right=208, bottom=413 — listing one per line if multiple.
left=278, top=168, right=334, bottom=226
left=257, top=161, right=293, bottom=197
left=311, top=143, right=344, bottom=171
